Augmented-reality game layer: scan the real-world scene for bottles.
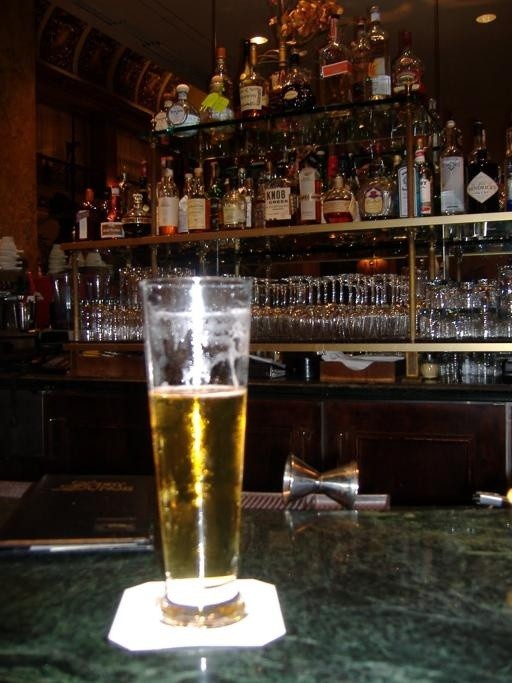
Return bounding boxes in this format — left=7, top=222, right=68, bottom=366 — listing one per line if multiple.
left=75, top=107, right=511, bottom=245
left=73, top=5, right=512, bottom=249
left=145, top=5, right=433, bottom=147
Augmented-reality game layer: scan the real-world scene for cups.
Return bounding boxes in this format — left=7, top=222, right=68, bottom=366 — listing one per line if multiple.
left=139, top=275, right=254, bottom=630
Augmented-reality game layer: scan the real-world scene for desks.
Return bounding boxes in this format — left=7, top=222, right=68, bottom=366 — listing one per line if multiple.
left=0, top=479, right=512, bottom=682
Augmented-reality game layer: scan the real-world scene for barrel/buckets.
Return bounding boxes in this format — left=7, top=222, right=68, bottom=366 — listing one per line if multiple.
left=7, top=302, right=32, bottom=332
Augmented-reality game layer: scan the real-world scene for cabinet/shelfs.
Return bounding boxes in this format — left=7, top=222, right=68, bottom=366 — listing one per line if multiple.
left=58, top=86, right=512, bottom=378
left=42, top=392, right=154, bottom=474
left=246, top=383, right=512, bottom=512
left=34, top=0, right=191, bottom=136
